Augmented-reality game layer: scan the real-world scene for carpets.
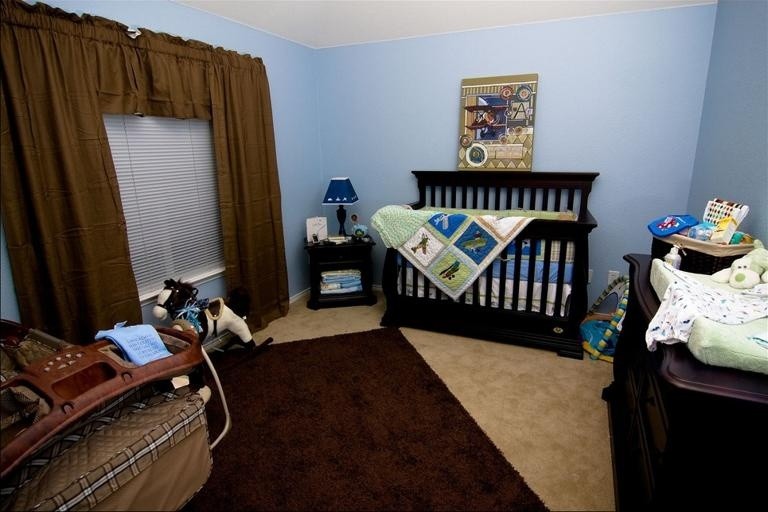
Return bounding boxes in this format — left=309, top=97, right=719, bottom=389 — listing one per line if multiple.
left=178, top=324, right=553, bottom=512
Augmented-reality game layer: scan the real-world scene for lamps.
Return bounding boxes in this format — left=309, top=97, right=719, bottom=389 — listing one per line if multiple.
left=317, top=177, right=361, bottom=239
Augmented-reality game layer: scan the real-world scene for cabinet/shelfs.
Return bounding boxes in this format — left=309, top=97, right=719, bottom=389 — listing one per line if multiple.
left=600, top=250, right=768, bottom=511
left=302, top=235, right=376, bottom=313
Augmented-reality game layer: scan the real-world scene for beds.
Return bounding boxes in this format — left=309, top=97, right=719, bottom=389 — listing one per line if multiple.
left=377, top=166, right=603, bottom=361
left=2, top=319, right=218, bottom=512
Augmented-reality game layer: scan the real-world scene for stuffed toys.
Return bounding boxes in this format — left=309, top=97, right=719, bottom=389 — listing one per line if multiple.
left=711, top=239, right=768, bottom=289
